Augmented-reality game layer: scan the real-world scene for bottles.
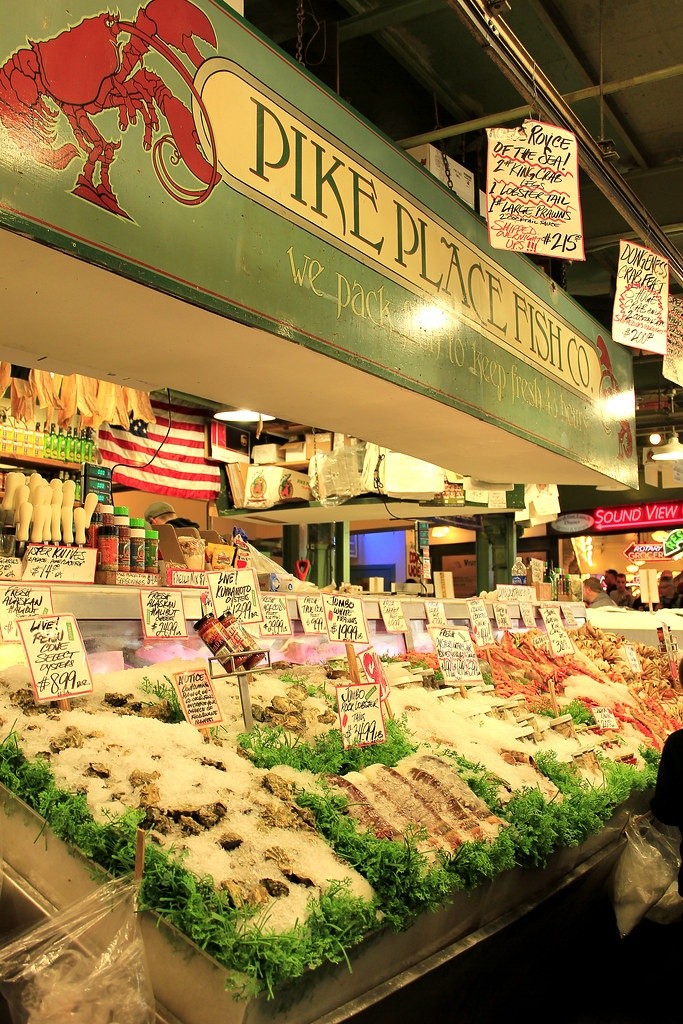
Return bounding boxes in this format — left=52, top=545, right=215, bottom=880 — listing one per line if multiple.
left=657, top=626, right=674, bottom=651
left=193, top=613, right=248, bottom=672
left=86, top=505, right=160, bottom=574
left=543, top=560, right=583, bottom=602
left=0, top=413, right=97, bottom=465
left=511, top=556, right=527, bottom=584
left=218, top=611, right=265, bottom=671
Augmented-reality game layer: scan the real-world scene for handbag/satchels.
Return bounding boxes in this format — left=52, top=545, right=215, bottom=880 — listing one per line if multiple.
left=614, top=815, right=683, bottom=938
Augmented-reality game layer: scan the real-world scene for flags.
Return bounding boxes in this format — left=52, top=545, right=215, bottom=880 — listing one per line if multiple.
left=98, top=400, right=222, bottom=499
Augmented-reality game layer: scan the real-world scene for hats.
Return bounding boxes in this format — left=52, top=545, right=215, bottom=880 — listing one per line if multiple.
left=144, top=502, right=175, bottom=522
left=661, top=570, right=672, bottom=577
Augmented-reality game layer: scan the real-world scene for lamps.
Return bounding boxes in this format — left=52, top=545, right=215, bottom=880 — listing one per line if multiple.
left=648, top=388, right=683, bottom=460
left=431, top=526, right=450, bottom=537
left=213, top=405, right=276, bottom=422
left=384, top=449, right=444, bottom=500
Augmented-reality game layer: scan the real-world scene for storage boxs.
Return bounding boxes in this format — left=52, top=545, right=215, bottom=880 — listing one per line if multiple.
left=441, top=551, right=553, bottom=602
left=204, top=417, right=361, bottom=510
left=404, top=142, right=476, bottom=210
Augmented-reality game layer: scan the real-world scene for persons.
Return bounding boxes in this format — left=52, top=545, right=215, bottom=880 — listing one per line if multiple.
left=582, top=569, right=633, bottom=609
left=650, top=662, right=683, bottom=897
left=146, top=502, right=199, bottom=529
left=633, top=570, right=683, bottom=611
